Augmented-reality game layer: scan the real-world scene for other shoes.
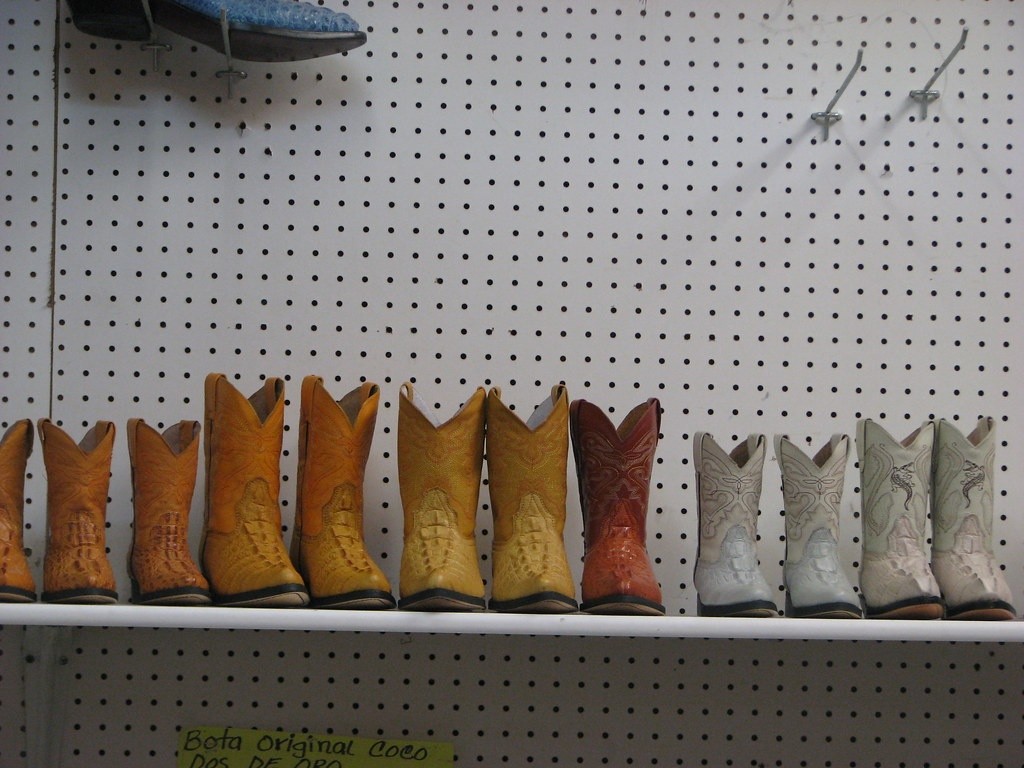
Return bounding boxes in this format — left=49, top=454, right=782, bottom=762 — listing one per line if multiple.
left=64, top=0, right=367, bottom=63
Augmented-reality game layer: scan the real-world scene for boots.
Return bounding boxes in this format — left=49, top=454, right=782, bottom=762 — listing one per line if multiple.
left=398, top=381, right=487, bottom=611
left=487, top=383, right=577, bottom=613
left=288, top=374, right=397, bottom=609
left=774, top=432, right=863, bottom=618
left=930, top=418, right=1018, bottom=619
left=569, top=397, right=665, bottom=616
left=854, top=417, right=944, bottom=620
left=692, top=431, right=778, bottom=618
left=198, top=374, right=309, bottom=607
left=127, top=416, right=211, bottom=605
left=0, top=418, right=38, bottom=602
left=36, top=419, right=120, bottom=603
